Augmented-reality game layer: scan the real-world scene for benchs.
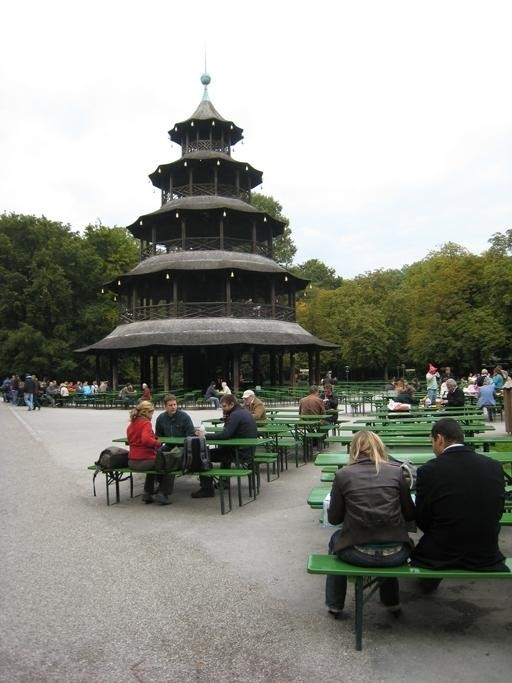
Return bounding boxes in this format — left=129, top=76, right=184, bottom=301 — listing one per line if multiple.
left=86, top=407, right=350, bottom=513
left=13, top=374, right=512, bottom=410
left=308, top=404, right=512, bottom=649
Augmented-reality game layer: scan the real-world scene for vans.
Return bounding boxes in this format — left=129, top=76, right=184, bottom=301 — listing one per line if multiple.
left=298, top=368, right=310, bottom=378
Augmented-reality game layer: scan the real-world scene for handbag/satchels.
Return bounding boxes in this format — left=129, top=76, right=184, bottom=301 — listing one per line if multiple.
left=94, top=447, right=129, bottom=468
left=388, top=454, right=418, bottom=491
left=156, top=446, right=184, bottom=472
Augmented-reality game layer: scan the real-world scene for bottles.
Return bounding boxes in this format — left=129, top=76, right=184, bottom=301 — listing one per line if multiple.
left=200, top=423, right=205, bottom=438
left=425, top=397, right=431, bottom=407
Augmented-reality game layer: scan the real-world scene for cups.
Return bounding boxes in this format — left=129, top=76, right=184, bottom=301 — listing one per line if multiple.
left=420, top=399, right=424, bottom=407
left=436, top=399, right=441, bottom=409
left=323, top=498, right=332, bottom=526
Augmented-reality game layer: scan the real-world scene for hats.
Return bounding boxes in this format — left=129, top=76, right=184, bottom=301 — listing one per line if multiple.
left=428, top=363, right=438, bottom=372
left=242, top=389, right=255, bottom=398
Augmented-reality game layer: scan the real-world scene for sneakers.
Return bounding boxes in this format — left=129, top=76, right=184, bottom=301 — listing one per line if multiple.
left=154, top=494, right=172, bottom=505
left=192, top=488, right=214, bottom=498
left=142, top=494, right=153, bottom=502
left=214, top=482, right=228, bottom=489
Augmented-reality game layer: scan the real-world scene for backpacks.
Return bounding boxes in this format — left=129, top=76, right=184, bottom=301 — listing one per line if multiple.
left=183, top=436, right=210, bottom=472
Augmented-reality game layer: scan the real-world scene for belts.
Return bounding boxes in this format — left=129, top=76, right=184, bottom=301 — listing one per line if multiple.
left=353, top=545, right=403, bottom=556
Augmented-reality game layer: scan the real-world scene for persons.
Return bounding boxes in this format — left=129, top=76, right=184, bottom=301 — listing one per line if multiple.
left=152, top=394, right=196, bottom=494
left=205, top=381, right=222, bottom=410
left=414, top=418, right=510, bottom=590
left=0, top=373, right=150, bottom=411
left=386, top=362, right=512, bottom=421
left=127, top=401, right=174, bottom=504
left=241, top=390, right=266, bottom=437
left=191, top=395, right=257, bottom=498
left=290, top=376, right=337, bottom=446
left=218, top=381, right=231, bottom=408
left=325, top=430, right=416, bottom=619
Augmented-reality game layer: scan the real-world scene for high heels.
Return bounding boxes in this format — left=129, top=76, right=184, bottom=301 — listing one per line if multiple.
left=386, top=605, right=401, bottom=618
left=327, top=607, right=342, bottom=619
